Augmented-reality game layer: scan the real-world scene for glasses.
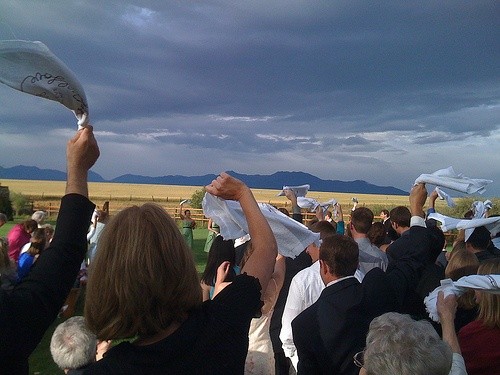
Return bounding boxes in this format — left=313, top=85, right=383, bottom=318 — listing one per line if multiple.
left=353, top=347, right=366, bottom=369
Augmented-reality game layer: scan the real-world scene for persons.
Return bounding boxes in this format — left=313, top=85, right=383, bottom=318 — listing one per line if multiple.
left=0, top=171, right=500, bottom=375
left=0, top=124, right=101, bottom=375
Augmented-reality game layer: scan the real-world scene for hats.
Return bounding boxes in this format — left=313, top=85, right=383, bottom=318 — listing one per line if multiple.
left=31, top=211, right=46, bottom=229
